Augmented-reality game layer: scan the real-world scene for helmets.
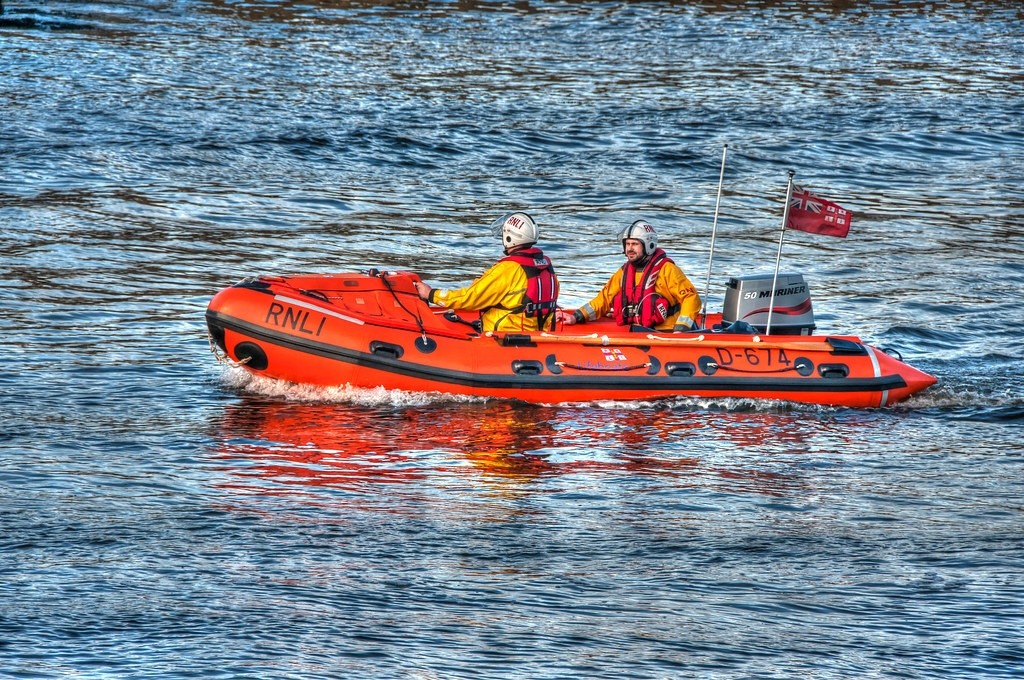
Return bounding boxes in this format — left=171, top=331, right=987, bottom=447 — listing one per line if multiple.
left=501, top=212, right=538, bottom=247
left=623, top=220, right=657, bottom=255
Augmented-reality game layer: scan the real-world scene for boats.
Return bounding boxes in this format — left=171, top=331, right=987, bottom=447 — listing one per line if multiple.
left=205, top=145, right=939, bottom=408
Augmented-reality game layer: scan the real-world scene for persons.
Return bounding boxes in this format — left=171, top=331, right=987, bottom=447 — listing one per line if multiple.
left=415, top=212, right=560, bottom=332
left=556, top=220, right=702, bottom=333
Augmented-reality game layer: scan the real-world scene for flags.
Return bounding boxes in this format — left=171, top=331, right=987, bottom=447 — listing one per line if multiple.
left=786, top=185, right=851, bottom=238
